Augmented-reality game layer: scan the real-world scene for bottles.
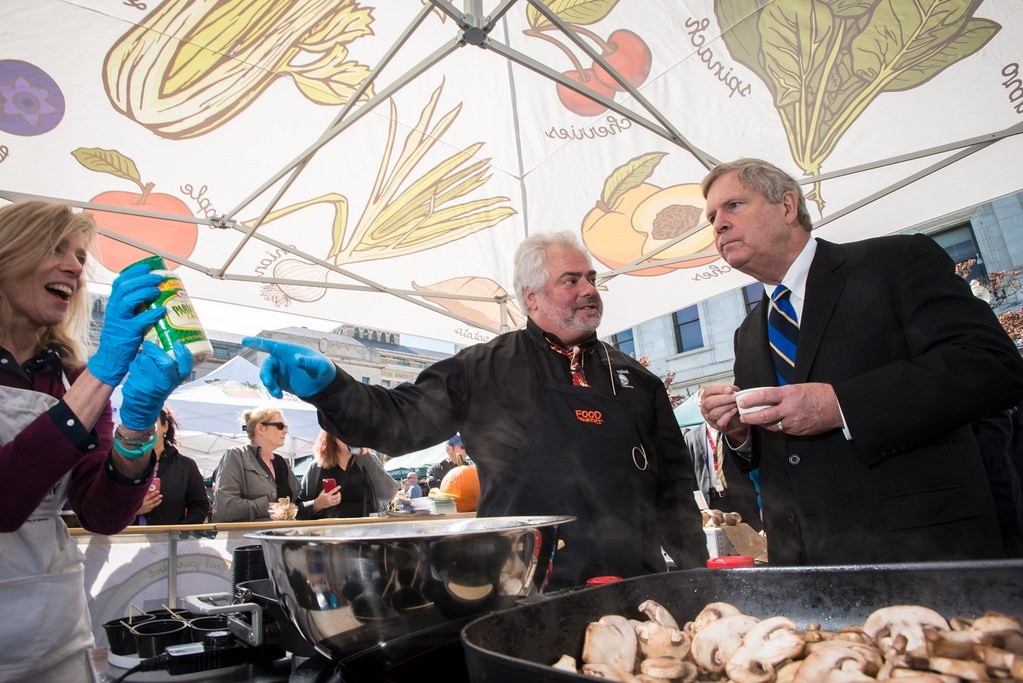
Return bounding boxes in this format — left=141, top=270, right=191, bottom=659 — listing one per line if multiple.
left=119, top=255, right=214, bottom=367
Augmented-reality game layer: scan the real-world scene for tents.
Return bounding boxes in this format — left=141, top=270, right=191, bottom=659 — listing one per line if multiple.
left=164, top=354, right=322, bottom=478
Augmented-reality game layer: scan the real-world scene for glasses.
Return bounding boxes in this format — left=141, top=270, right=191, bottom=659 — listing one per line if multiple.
left=261, top=422, right=288, bottom=430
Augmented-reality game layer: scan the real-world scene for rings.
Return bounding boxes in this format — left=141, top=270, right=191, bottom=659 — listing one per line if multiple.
left=777, top=421, right=783, bottom=431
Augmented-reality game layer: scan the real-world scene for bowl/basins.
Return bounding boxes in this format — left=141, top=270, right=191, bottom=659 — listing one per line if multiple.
left=734, top=387, right=776, bottom=414
left=102, top=608, right=228, bottom=659
left=461, top=563, right=1023, bottom=683
left=268, top=507, right=298, bottom=520
left=244, top=513, right=583, bottom=670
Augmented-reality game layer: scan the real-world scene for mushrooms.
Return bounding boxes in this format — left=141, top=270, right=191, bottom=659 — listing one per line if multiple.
left=555, top=600, right=1023, bottom=683
left=699, top=508, right=742, bottom=527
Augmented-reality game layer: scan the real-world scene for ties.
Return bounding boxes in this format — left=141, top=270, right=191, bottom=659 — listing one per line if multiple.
left=546, top=338, right=591, bottom=387
left=767, top=283, right=800, bottom=385
left=706, top=427, right=728, bottom=487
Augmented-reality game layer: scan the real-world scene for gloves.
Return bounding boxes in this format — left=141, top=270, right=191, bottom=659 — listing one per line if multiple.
left=242, top=336, right=336, bottom=399
left=88, top=263, right=167, bottom=387
left=118, top=340, right=193, bottom=431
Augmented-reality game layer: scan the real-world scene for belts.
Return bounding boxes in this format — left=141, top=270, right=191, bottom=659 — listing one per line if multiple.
left=712, top=491, right=727, bottom=499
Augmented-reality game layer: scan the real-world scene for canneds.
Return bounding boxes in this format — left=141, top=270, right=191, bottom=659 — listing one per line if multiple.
left=119, top=256, right=213, bottom=369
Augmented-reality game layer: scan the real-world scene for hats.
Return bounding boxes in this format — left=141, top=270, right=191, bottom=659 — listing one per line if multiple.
left=448, top=435, right=463, bottom=445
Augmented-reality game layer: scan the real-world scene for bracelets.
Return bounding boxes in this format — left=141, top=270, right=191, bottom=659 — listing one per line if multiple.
left=113, top=424, right=157, bottom=459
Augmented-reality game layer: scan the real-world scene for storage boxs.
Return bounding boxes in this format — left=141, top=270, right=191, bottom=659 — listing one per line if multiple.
left=660, top=529, right=733, bottom=564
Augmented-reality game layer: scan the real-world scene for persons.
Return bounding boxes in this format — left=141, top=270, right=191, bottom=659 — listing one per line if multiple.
left=242, top=231, right=711, bottom=592
left=132, top=409, right=209, bottom=526
left=683, top=388, right=763, bottom=533
left=212, top=407, right=301, bottom=523
left=1, top=199, right=194, bottom=683
left=302, top=429, right=399, bottom=518
left=426, top=436, right=468, bottom=489
left=698, top=158, right=1023, bottom=566
left=395, top=472, right=422, bottom=511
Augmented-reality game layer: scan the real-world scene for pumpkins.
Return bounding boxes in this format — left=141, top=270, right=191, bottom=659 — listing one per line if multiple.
left=440, top=453, right=482, bottom=512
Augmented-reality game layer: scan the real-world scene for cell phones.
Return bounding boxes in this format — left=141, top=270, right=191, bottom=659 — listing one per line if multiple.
left=322, top=478, right=338, bottom=495
left=147, top=477, right=161, bottom=494
